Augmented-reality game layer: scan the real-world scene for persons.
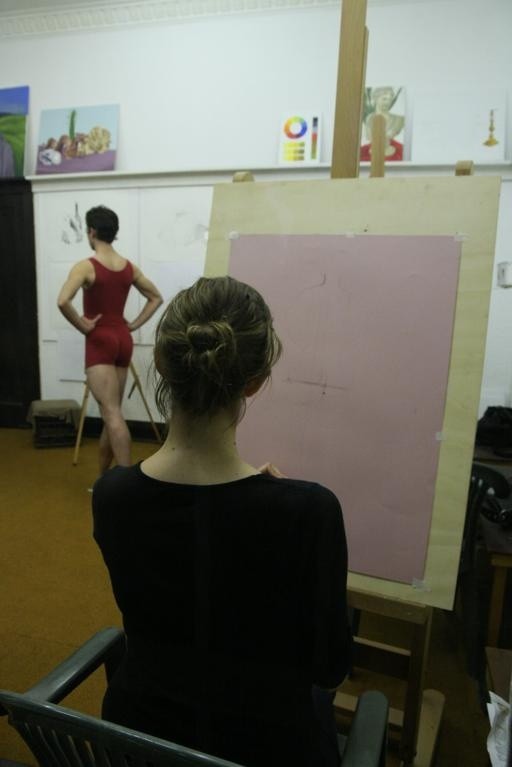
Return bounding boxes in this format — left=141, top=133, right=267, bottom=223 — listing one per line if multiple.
left=93, top=276, right=353, bottom=767
left=56, top=204, right=164, bottom=490
left=366, top=87, right=405, bottom=156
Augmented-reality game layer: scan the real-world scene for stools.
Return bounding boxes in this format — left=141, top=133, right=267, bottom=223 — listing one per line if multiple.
left=31, top=398, right=83, bottom=448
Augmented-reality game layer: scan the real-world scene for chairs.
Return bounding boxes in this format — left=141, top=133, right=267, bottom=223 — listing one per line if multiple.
left=0, top=626, right=390, bottom=765
